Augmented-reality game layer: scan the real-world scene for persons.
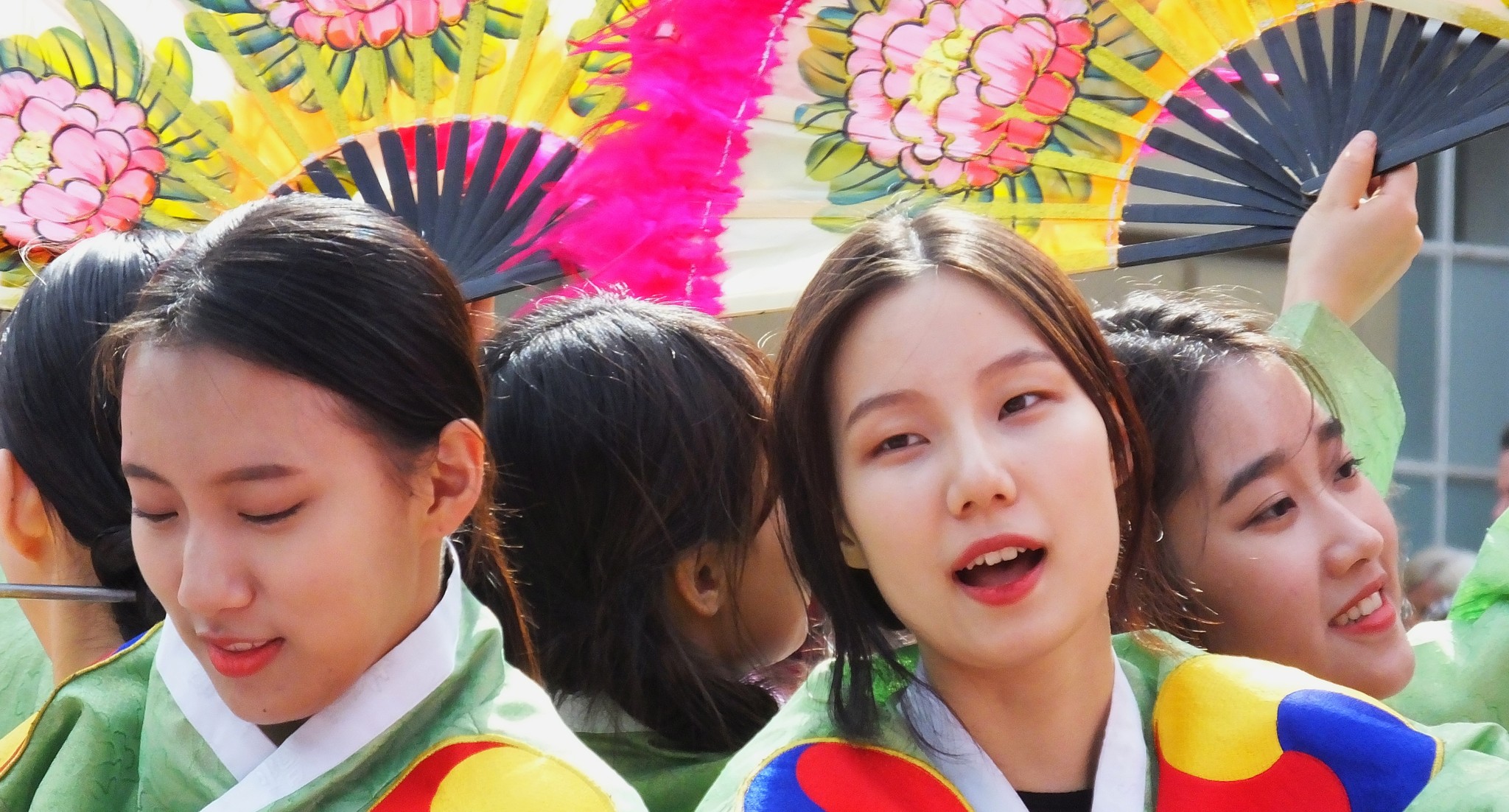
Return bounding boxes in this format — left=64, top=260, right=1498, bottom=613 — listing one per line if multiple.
left=1079, top=281, right=1417, bottom=701
left=0, top=229, right=184, bottom=781
left=475, top=286, right=807, bottom=812
left=0, top=130, right=1509, bottom=812
left=692, top=202, right=1509, bottom=812
